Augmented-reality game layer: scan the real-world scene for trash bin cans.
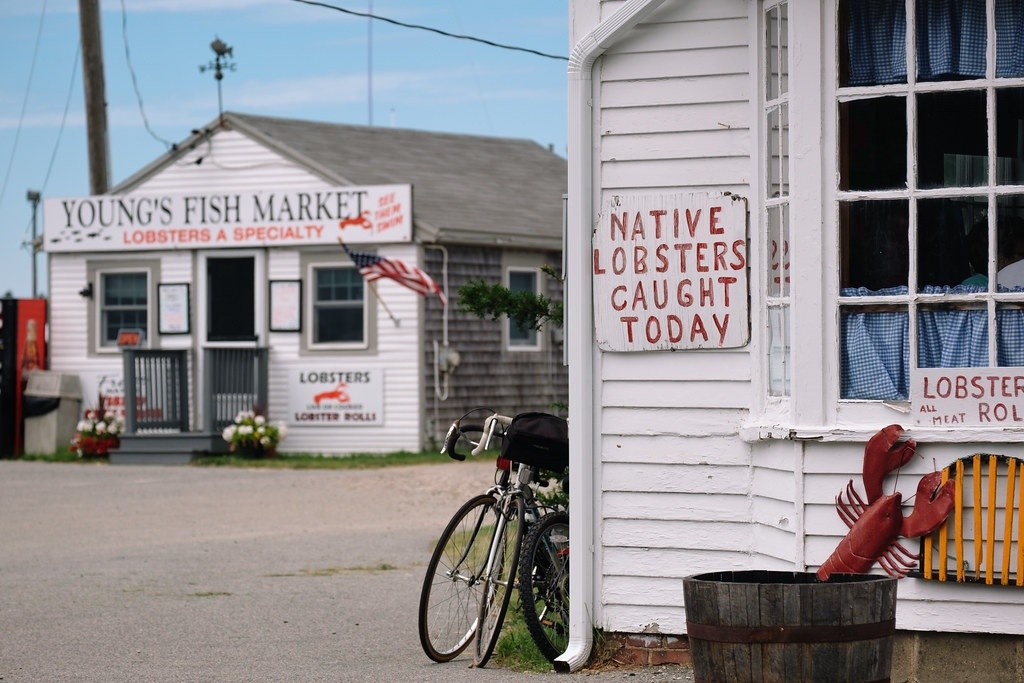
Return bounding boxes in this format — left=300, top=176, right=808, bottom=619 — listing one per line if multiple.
left=24, top=371, right=83, bottom=456
left=682, top=570, right=897, bottom=683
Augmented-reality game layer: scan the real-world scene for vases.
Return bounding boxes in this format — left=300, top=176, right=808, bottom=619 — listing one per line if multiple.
left=80, top=435, right=119, bottom=454
left=228, top=440, right=275, bottom=457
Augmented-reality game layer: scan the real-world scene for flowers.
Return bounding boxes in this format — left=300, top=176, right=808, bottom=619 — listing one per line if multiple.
left=76, top=410, right=123, bottom=437
left=222, top=411, right=286, bottom=449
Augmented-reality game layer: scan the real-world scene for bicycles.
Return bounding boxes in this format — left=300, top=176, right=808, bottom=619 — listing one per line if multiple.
left=417, top=406, right=568, bottom=671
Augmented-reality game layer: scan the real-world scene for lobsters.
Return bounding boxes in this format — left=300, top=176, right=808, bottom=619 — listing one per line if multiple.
left=816, top=425, right=957, bottom=584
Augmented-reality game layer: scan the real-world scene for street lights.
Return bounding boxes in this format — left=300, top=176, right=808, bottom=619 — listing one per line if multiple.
left=25, top=188, right=43, bottom=300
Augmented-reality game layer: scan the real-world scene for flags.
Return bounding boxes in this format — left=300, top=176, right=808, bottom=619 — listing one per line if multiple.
left=343, top=243, right=447, bottom=305
